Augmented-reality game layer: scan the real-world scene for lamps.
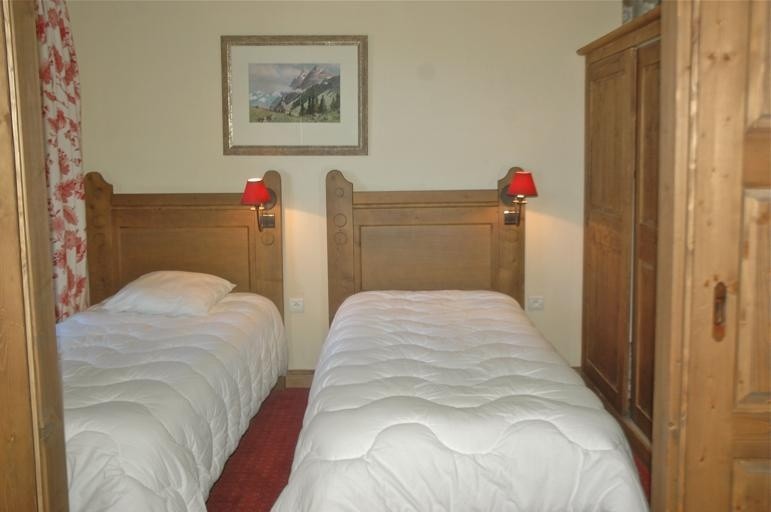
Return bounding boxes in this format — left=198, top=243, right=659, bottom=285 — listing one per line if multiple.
left=504, top=171, right=538, bottom=226
left=241, top=178, right=275, bottom=232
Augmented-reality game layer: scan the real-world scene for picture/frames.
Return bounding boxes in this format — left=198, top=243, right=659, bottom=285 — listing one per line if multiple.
left=221, top=35, right=368, bottom=156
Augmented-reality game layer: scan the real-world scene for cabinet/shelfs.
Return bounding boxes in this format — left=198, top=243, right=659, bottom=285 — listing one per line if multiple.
left=576, top=5, right=660, bottom=469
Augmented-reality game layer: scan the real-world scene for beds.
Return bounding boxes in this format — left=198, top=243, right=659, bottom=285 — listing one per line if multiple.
left=56, top=170, right=288, bottom=512
left=271, top=168, right=649, bottom=512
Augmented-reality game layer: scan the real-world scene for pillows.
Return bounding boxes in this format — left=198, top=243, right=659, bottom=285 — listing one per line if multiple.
left=101, top=270, right=237, bottom=317
